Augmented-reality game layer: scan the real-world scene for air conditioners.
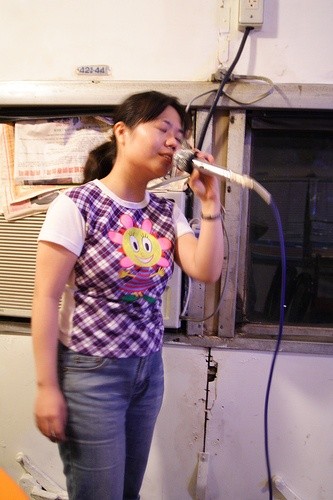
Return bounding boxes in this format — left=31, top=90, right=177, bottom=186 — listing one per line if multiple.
left=0, top=192, right=186, bottom=328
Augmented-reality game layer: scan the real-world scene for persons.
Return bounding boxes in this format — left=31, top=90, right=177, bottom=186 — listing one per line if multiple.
left=30, top=90, right=221, bottom=500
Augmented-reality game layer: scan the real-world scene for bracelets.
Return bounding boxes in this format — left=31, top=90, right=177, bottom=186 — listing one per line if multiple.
left=199, top=210, right=221, bottom=221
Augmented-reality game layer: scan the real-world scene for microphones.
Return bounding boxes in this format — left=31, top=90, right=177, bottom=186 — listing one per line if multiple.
left=172, top=148, right=255, bottom=189
